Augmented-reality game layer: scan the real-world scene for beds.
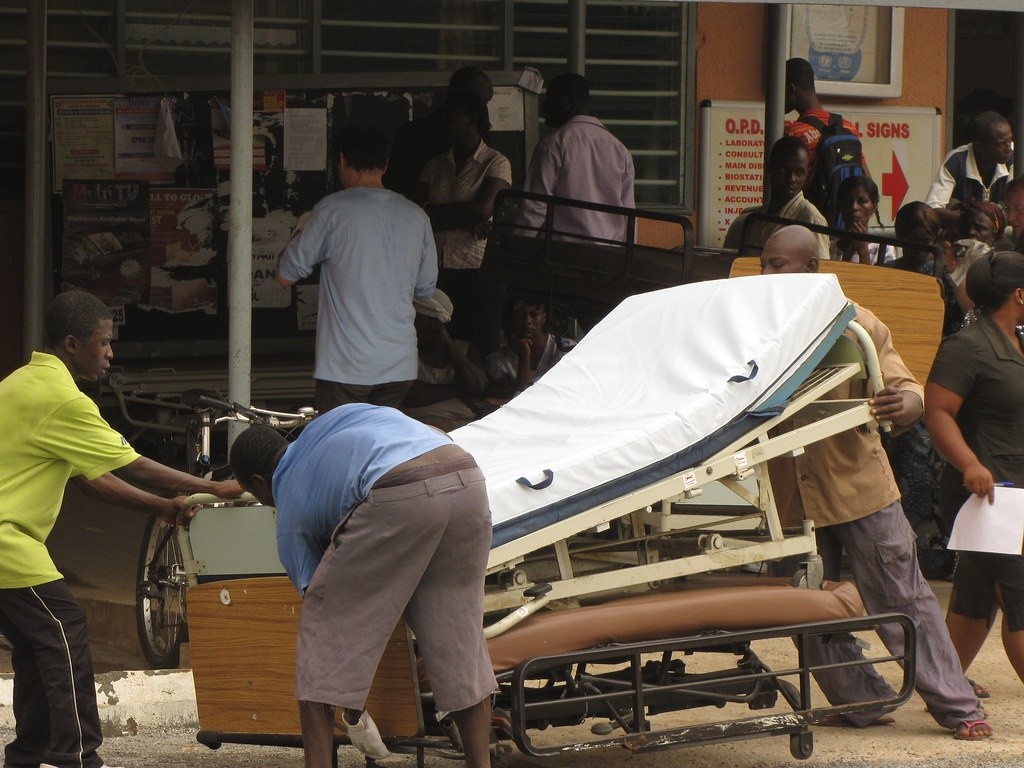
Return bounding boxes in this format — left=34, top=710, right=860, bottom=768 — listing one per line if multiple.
left=184, top=576, right=920, bottom=768
left=177, top=268, right=892, bottom=638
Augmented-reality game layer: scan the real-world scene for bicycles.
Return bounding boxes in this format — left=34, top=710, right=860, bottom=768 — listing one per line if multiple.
left=135, top=393, right=319, bottom=667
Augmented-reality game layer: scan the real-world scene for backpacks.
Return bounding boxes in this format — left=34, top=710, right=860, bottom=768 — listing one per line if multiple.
left=798, top=113, right=866, bottom=231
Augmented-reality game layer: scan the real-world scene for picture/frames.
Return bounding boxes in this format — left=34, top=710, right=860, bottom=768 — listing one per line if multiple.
left=779, top=2, right=907, bottom=100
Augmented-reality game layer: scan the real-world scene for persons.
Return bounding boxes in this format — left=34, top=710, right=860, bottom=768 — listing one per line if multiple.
left=835, top=175, right=896, bottom=266
left=759, top=225, right=996, bottom=740
left=480, top=289, right=578, bottom=395
left=784, top=56, right=872, bottom=222
left=0, top=289, right=247, bottom=768
left=399, top=284, right=489, bottom=408
left=229, top=402, right=501, bottom=768
left=887, top=110, right=1024, bottom=701
left=510, top=68, right=635, bottom=250
left=386, top=65, right=499, bottom=202
left=723, top=134, right=831, bottom=261
left=409, top=94, right=513, bottom=275
left=276, top=125, right=439, bottom=413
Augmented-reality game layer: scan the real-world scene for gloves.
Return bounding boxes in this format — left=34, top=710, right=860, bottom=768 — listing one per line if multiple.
left=342, top=710, right=391, bottom=760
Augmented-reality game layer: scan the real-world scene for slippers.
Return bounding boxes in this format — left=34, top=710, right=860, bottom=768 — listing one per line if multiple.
left=813, top=713, right=896, bottom=727
left=967, top=679, right=990, bottom=697
left=952, top=720, right=993, bottom=740
